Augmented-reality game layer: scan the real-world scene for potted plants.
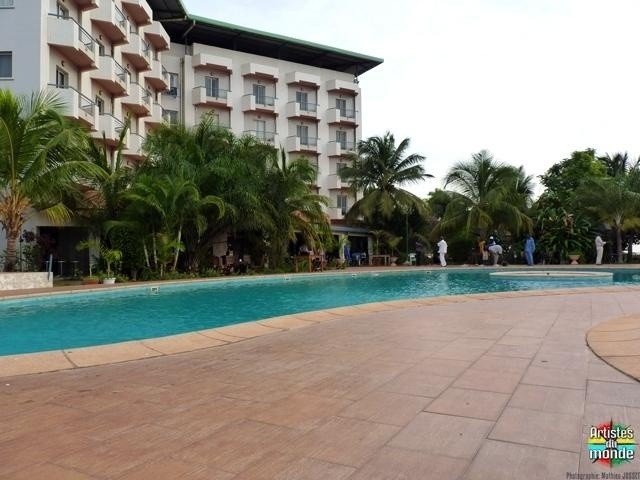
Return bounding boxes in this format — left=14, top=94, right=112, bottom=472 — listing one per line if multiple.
left=99, top=270, right=117, bottom=285
left=567, top=251, right=583, bottom=264
left=390, top=255, right=400, bottom=266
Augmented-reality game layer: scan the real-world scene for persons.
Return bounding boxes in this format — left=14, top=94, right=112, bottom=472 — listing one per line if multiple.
left=414, top=236, right=424, bottom=267
left=484, top=236, right=496, bottom=250
left=523, top=232, right=536, bottom=267
left=488, top=244, right=506, bottom=266
left=471, top=241, right=481, bottom=266
left=594, top=232, right=610, bottom=265
left=436, top=236, right=448, bottom=267
